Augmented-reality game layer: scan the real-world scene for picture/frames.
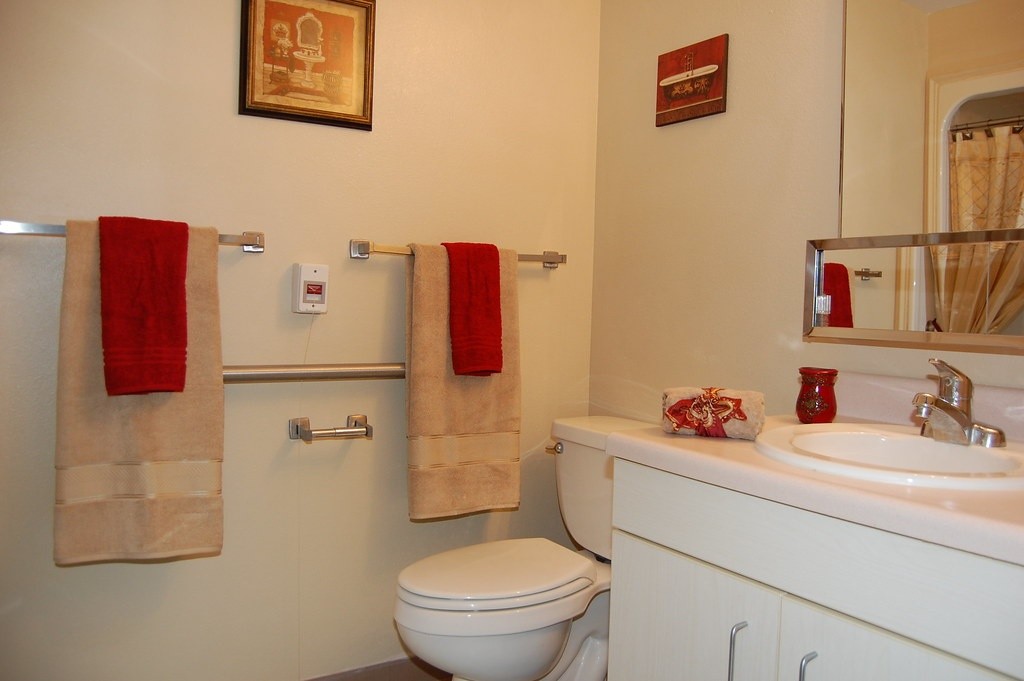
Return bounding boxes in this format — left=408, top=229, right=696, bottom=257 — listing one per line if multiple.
left=238, top=0, right=377, bottom=131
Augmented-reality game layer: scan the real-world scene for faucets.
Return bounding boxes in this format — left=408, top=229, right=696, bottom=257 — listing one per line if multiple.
left=911, top=359, right=1005, bottom=449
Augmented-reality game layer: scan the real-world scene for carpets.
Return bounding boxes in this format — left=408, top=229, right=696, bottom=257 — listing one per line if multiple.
left=268, top=86, right=346, bottom=105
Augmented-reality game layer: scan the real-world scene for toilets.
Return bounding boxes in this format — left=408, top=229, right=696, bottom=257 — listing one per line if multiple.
left=390, top=413, right=699, bottom=681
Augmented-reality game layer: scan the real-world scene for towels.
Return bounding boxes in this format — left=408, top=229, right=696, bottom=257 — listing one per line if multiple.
left=52, top=215, right=224, bottom=568
left=822, top=262, right=854, bottom=328
left=405, top=242, right=523, bottom=523
left=662, top=387, right=765, bottom=440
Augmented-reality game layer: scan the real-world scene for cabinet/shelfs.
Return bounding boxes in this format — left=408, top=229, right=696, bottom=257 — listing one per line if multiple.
left=608, top=457, right=1024, bottom=681
left=269, top=50, right=291, bottom=86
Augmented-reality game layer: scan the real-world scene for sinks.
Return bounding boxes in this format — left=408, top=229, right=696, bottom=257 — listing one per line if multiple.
left=756, top=422, right=1024, bottom=491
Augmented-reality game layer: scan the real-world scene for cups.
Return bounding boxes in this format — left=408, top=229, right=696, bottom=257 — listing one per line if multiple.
left=796, top=367, right=838, bottom=424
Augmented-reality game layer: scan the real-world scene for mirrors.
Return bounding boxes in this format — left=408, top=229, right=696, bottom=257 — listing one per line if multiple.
left=803, top=228, right=1024, bottom=356
left=839, top=0, right=1024, bottom=238
